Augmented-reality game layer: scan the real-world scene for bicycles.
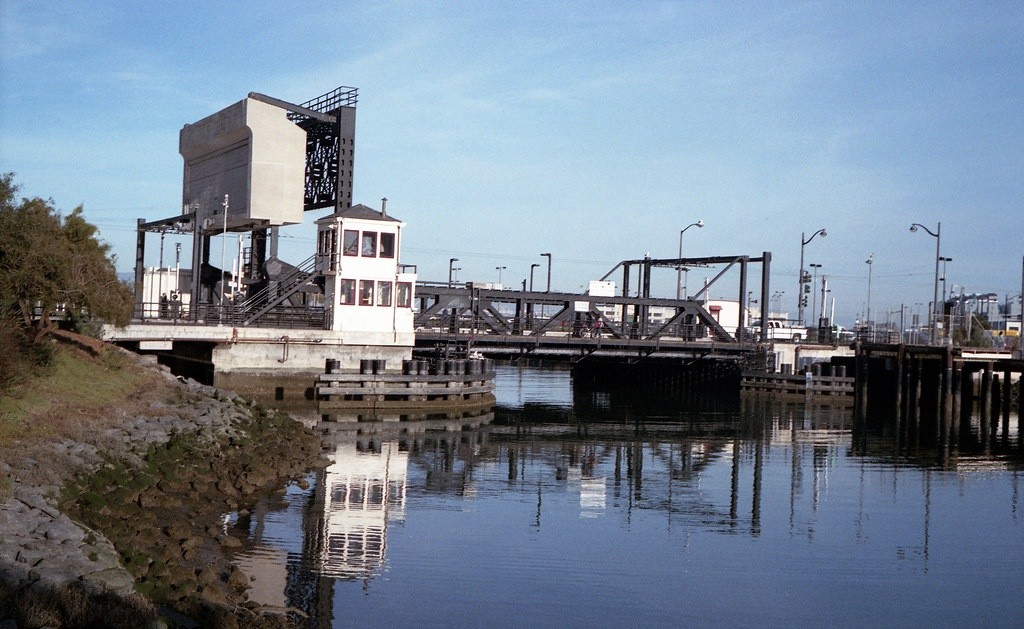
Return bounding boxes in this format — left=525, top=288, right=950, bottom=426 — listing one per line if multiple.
left=579, top=311, right=603, bottom=339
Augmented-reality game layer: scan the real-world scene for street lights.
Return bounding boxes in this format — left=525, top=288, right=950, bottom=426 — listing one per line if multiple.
left=674, top=264, right=691, bottom=274
left=770, top=291, right=785, bottom=303
left=748, top=290, right=754, bottom=297
left=496, top=264, right=507, bottom=291
left=529, top=262, right=542, bottom=292
left=937, top=274, right=951, bottom=283
left=938, top=256, right=955, bottom=264
left=1007, top=294, right=1022, bottom=300
left=751, top=298, right=759, bottom=303
left=544, top=251, right=552, bottom=289
left=863, top=258, right=873, bottom=319
left=798, top=228, right=827, bottom=329
left=676, top=219, right=705, bottom=299
left=909, top=223, right=945, bottom=314
left=811, top=262, right=823, bottom=333
left=448, top=256, right=460, bottom=294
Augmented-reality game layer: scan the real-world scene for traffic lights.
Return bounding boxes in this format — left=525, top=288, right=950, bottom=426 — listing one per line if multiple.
left=521, top=278, right=527, bottom=294
left=801, top=269, right=810, bottom=283
left=800, top=295, right=808, bottom=308
left=825, top=288, right=833, bottom=295
left=804, top=286, right=811, bottom=294
left=219, top=193, right=231, bottom=214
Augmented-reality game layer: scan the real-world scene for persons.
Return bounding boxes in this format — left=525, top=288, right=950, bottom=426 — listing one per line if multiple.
left=573, top=311, right=604, bottom=338
left=998, top=331, right=1005, bottom=349
left=161, top=293, right=169, bottom=317
